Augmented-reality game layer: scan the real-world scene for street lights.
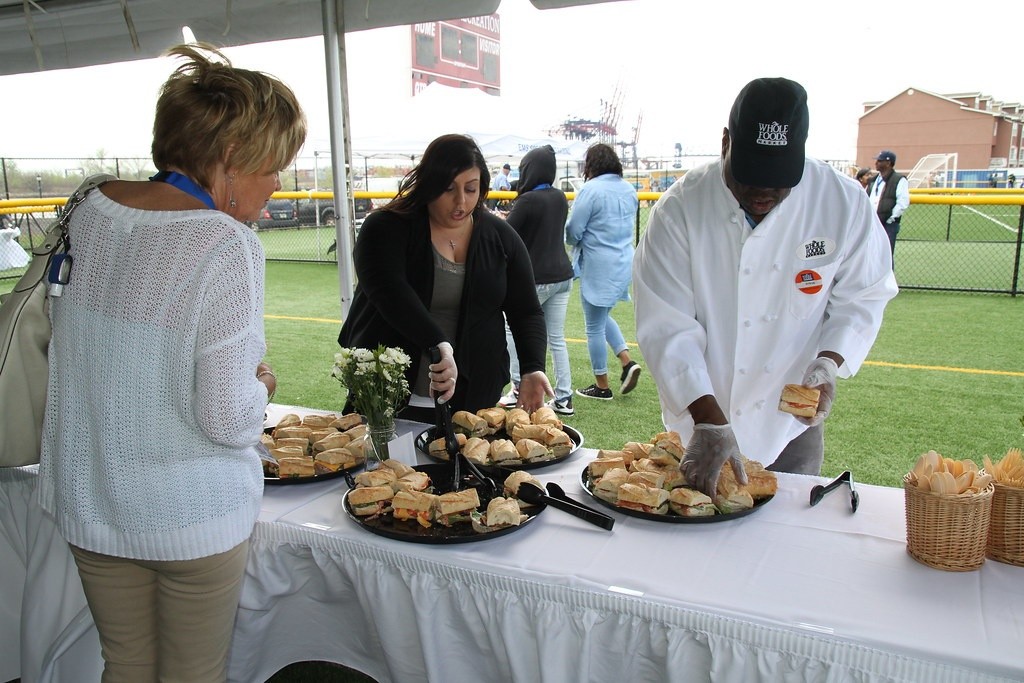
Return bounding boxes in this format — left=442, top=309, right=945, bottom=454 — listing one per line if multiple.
left=35, top=173, right=44, bottom=218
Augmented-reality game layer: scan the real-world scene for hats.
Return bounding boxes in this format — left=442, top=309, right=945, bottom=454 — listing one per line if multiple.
left=503, top=164, right=511, bottom=170
left=873, top=150, right=896, bottom=163
left=730, top=78, right=809, bottom=188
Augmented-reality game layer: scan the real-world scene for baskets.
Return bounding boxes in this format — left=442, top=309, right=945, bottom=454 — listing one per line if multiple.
left=903, top=468, right=1024, bottom=572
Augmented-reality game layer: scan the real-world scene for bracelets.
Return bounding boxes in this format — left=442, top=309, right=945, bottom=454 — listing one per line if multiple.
left=258, top=372, right=276, bottom=405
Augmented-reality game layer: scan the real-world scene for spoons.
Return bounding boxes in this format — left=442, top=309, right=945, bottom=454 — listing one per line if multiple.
left=908, top=450, right=992, bottom=496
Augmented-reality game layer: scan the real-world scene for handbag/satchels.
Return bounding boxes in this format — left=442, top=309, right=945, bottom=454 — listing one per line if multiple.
left=0, top=174, right=120, bottom=468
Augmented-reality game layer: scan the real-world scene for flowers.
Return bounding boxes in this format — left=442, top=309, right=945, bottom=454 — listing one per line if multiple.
left=331, top=341, right=413, bottom=462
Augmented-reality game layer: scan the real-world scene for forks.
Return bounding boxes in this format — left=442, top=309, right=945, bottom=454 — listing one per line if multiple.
left=983, top=448, right=1024, bottom=488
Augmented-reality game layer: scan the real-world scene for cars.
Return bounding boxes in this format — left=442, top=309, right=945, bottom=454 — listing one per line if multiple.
left=556, top=173, right=677, bottom=194
left=241, top=191, right=375, bottom=231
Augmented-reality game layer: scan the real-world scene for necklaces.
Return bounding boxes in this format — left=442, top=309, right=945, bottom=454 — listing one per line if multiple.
left=433, top=219, right=467, bottom=251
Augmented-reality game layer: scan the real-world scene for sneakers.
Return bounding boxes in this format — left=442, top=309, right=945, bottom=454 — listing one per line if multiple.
left=498, top=389, right=520, bottom=408
left=576, top=383, right=613, bottom=401
left=544, top=396, right=574, bottom=417
left=620, top=361, right=641, bottom=394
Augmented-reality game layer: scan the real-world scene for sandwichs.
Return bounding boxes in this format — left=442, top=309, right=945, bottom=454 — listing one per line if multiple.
left=260, top=413, right=373, bottom=479
left=586, top=432, right=778, bottom=517
left=347, top=459, right=544, bottom=533
left=428, top=406, right=574, bottom=468
left=777, top=384, right=821, bottom=418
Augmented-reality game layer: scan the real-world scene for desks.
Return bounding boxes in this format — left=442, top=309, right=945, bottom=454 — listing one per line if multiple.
left=0, top=403, right=1024, bottom=683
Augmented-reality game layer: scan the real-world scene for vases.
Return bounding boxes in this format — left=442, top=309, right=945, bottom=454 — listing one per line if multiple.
left=362, top=421, right=399, bottom=473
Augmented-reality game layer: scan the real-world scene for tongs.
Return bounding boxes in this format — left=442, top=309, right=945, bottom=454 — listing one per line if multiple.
left=450, top=452, right=497, bottom=497
left=515, top=482, right=615, bottom=531
left=810, top=470, right=859, bottom=513
left=429, top=347, right=460, bottom=458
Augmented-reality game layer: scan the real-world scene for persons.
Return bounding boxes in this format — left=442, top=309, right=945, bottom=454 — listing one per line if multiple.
left=496, top=145, right=574, bottom=415
left=989, top=173, right=998, bottom=188
left=862, top=151, right=910, bottom=272
left=336, top=134, right=556, bottom=427
left=1020, top=178, right=1024, bottom=188
left=856, top=168, right=872, bottom=190
left=491, top=163, right=512, bottom=211
left=564, top=144, right=642, bottom=401
left=631, top=77, right=900, bottom=502
left=1007, top=174, right=1015, bottom=188
left=32, top=45, right=307, bottom=683
left=929, top=172, right=941, bottom=187
